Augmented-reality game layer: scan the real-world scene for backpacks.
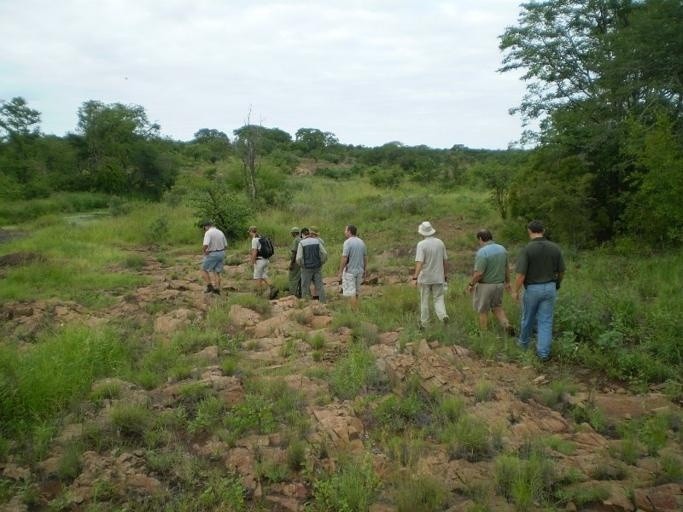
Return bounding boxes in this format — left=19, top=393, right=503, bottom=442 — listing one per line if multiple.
left=257, top=236, right=273, bottom=259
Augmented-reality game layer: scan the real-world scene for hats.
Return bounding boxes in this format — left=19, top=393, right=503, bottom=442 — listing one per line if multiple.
left=290, top=226, right=318, bottom=238
left=417, top=222, right=435, bottom=236
left=247, top=226, right=256, bottom=232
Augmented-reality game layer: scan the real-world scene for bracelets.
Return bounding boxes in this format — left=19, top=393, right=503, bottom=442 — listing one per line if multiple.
left=412, top=278, right=417, bottom=280
left=469, top=282, right=474, bottom=286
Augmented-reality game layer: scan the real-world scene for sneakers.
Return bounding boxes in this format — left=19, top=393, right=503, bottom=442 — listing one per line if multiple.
left=203, top=285, right=219, bottom=295
left=269, top=287, right=278, bottom=299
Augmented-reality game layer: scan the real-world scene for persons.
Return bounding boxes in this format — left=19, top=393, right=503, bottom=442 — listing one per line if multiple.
left=248, top=225, right=279, bottom=299
left=289, top=226, right=304, bottom=299
left=410, top=220, right=449, bottom=329
left=309, top=226, right=324, bottom=247
left=295, top=228, right=328, bottom=303
left=464, top=229, right=515, bottom=338
left=200, top=220, right=228, bottom=295
left=511, top=219, right=566, bottom=361
left=338, top=224, right=368, bottom=309
